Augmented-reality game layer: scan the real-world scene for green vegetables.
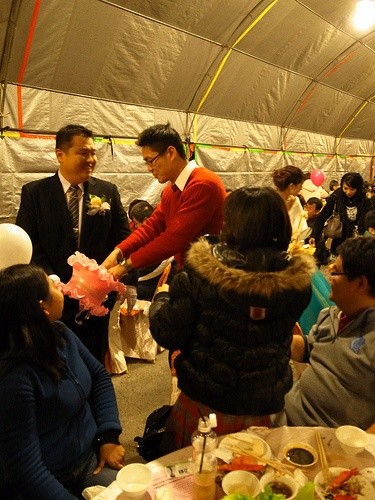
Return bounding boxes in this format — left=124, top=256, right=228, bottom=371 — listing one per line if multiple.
left=220, top=480, right=321, bottom=500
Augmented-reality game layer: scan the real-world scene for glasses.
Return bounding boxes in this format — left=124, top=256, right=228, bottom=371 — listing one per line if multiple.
left=140, top=151, right=164, bottom=167
left=304, top=205, right=313, bottom=212
left=329, top=258, right=345, bottom=275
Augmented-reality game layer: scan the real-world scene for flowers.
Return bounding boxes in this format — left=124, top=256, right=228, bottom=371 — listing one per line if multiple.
left=85, top=194, right=112, bottom=216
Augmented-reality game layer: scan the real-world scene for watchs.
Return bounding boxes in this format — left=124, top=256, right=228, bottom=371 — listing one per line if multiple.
left=120, top=258, right=130, bottom=273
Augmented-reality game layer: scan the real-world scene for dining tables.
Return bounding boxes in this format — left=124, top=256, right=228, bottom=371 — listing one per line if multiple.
left=87, top=427, right=375, bottom=500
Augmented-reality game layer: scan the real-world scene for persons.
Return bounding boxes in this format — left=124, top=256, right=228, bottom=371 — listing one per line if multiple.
left=122, top=198, right=165, bottom=302
left=284, top=237, right=375, bottom=432
left=150, top=187, right=314, bottom=451
left=14, top=124, right=130, bottom=364
left=271, top=165, right=308, bottom=245
left=104, top=122, right=226, bottom=375
left=0, top=265, right=125, bottom=500
left=299, top=171, right=375, bottom=268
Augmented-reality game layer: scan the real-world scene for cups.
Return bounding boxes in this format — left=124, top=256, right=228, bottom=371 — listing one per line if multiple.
left=116, top=463, right=153, bottom=500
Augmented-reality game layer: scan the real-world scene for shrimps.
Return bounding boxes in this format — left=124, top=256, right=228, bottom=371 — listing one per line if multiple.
left=324, top=466, right=361, bottom=492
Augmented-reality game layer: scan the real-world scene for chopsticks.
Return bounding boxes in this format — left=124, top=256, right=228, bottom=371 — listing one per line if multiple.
left=224, top=444, right=297, bottom=475
left=315, top=431, right=331, bottom=482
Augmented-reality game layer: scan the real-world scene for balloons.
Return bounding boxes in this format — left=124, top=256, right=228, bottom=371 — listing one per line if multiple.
left=0, top=223, right=33, bottom=271
left=310, top=169, right=325, bottom=186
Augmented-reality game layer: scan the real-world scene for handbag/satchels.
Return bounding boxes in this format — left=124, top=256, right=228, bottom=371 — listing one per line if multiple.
left=322, top=195, right=344, bottom=239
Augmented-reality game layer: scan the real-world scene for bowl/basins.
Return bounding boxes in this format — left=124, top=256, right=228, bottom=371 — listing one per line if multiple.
left=336, top=425, right=370, bottom=455
left=260, top=472, right=298, bottom=500
left=222, top=470, right=261, bottom=498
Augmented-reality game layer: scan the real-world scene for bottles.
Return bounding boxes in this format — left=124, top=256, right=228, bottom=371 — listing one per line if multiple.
left=191, top=417, right=217, bottom=487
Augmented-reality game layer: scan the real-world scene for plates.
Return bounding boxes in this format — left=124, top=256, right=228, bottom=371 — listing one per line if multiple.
left=218, top=432, right=271, bottom=466
left=361, top=467, right=375, bottom=493
left=363, top=433, right=375, bottom=457
left=283, top=442, right=318, bottom=467
left=313, top=466, right=375, bottom=500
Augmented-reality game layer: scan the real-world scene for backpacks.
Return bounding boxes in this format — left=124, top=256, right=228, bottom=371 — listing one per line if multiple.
left=133, top=404, right=175, bottom=453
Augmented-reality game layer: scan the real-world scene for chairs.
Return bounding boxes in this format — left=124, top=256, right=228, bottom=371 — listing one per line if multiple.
left=104, top=286, right=137, bottom=373
left=118, top=263, right=172, bottom=363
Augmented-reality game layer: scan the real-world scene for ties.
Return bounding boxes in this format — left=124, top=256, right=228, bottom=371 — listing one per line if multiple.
left=67, top=184, right=80, bottom=245
